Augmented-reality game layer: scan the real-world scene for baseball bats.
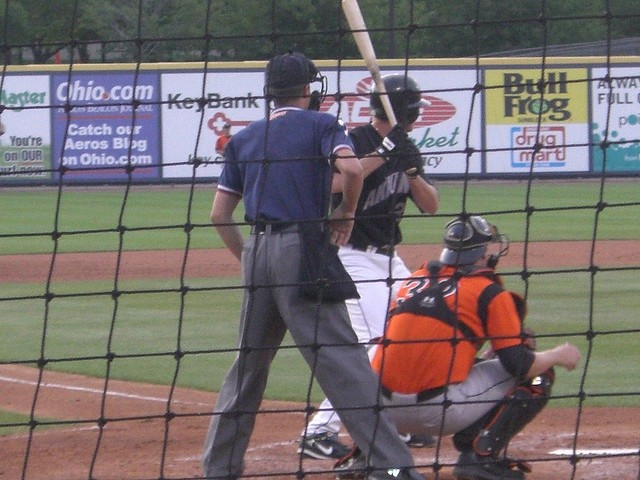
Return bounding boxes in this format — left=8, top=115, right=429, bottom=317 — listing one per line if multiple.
left=342, top=0, right=417, bottom=176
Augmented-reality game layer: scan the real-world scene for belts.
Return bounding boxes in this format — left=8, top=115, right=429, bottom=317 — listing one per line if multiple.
left=346, top=238, right=394, bottom=255
left=252, top=222, right=298, bottom=233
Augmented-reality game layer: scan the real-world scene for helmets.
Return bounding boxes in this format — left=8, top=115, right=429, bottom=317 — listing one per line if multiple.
left=263, top=48, right=328, bottom=111
left=371, top=74, right=432, bottom=114
left=439, top=215, right=509, bottom=269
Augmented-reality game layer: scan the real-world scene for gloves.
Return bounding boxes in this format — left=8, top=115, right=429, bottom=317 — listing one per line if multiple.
left=400, top=139, right=422, bottom=177
left=377, top=124, right=408, bottom=159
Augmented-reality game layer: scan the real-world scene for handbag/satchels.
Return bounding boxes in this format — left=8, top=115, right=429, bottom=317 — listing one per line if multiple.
left=300, top=227, right=360, bottom=301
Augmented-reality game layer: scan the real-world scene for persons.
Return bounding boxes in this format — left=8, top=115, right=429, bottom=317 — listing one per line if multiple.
left=214, top=123, right=236, bottom=181
left=203, top=50, right=429, bottom=479
left=370, top=214, right=581, bottom=480
left=296, top=73, right=440, bottom=463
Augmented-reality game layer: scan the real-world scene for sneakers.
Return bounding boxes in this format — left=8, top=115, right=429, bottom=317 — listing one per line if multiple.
left=368, top=465, right=429, bottom=479
left=297, top=433, right=351, bottom=461
left=453, top=447, right=532, bottom=479
left=334, top=451, right=372, bottom=480
left=397, top=432, right=436, bottom=449
